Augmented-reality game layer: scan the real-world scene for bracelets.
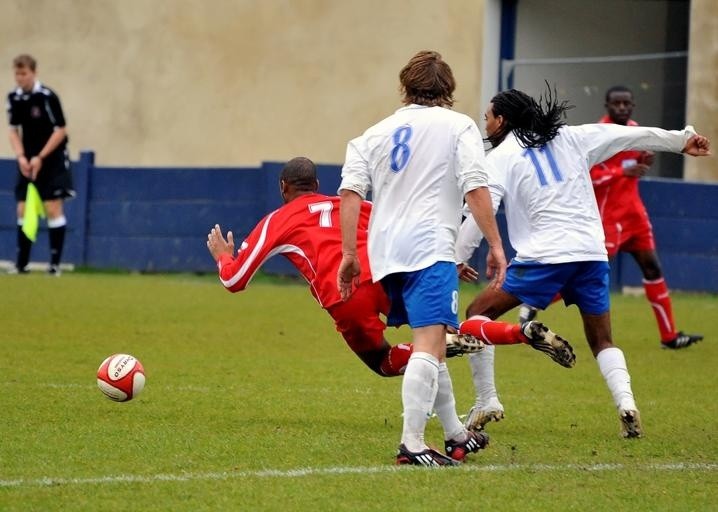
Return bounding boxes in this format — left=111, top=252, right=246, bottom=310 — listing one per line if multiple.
left=14, top=153, right=26, bottom=161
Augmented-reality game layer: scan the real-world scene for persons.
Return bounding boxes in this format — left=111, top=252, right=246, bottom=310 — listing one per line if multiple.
left=207, top=157, right=577, bottom=377
left=453, top=77, right=712, bottom=437
left=4, top=54, right=77, bottom=275
left=518, top=86, right=704, bottom=350
left=336, top=50, right=507, bottom=467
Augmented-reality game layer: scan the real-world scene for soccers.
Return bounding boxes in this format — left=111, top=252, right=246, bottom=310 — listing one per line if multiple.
left=97, top=354, right=147, bottom=402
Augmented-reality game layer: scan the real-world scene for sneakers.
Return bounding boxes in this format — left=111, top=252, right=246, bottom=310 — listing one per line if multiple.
left=518, top=303, right=539, bottom=325
left=522, top=319, right=577, bottom=371
left=443, top=428, right=491, bottom=462
left=618, top=408, right=646, bottom=442
left=462, top=396, right=506, bottom=432
left=445, top=331, right=487, bottom=360
left=395, top=442, right=464, bottom=468
left=660, top=331, right=707, bottom=352
